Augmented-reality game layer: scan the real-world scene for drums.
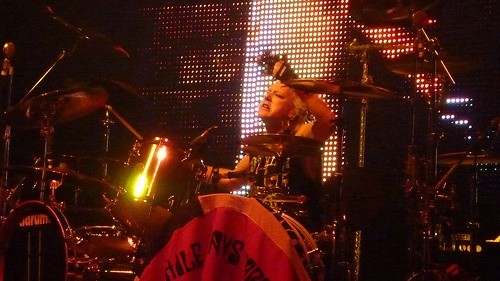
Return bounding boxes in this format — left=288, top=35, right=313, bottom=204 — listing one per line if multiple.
left=132, top=192, right=326, bottom=281
left=0, top=199, right=141, bottom=281
left=115, top=136, right=207, bottom=235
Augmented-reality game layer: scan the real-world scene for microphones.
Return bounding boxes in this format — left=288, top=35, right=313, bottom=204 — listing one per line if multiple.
left=190, top=124, right=216, bottom=145
left=420, top=27, right=456, bottom=87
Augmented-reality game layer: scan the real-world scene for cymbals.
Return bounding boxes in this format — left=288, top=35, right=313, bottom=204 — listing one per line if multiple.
left=8, top=163, right=124, bottom=194
left=45, top=5, right=129, bottom=57
left=351, top=0, right=439, bottom=25
left=7, top=86, right=109, bottom=127
left=287, top=78, right=398, bottom=103
left=385, top=52, right=483, bottom=74
left=243, top=133, right=323, bottom=154
left=437, top=151, right=500, bottom=166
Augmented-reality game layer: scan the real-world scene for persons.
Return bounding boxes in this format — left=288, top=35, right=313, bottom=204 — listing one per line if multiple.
left=192, top=49, right=337, bottom=240
